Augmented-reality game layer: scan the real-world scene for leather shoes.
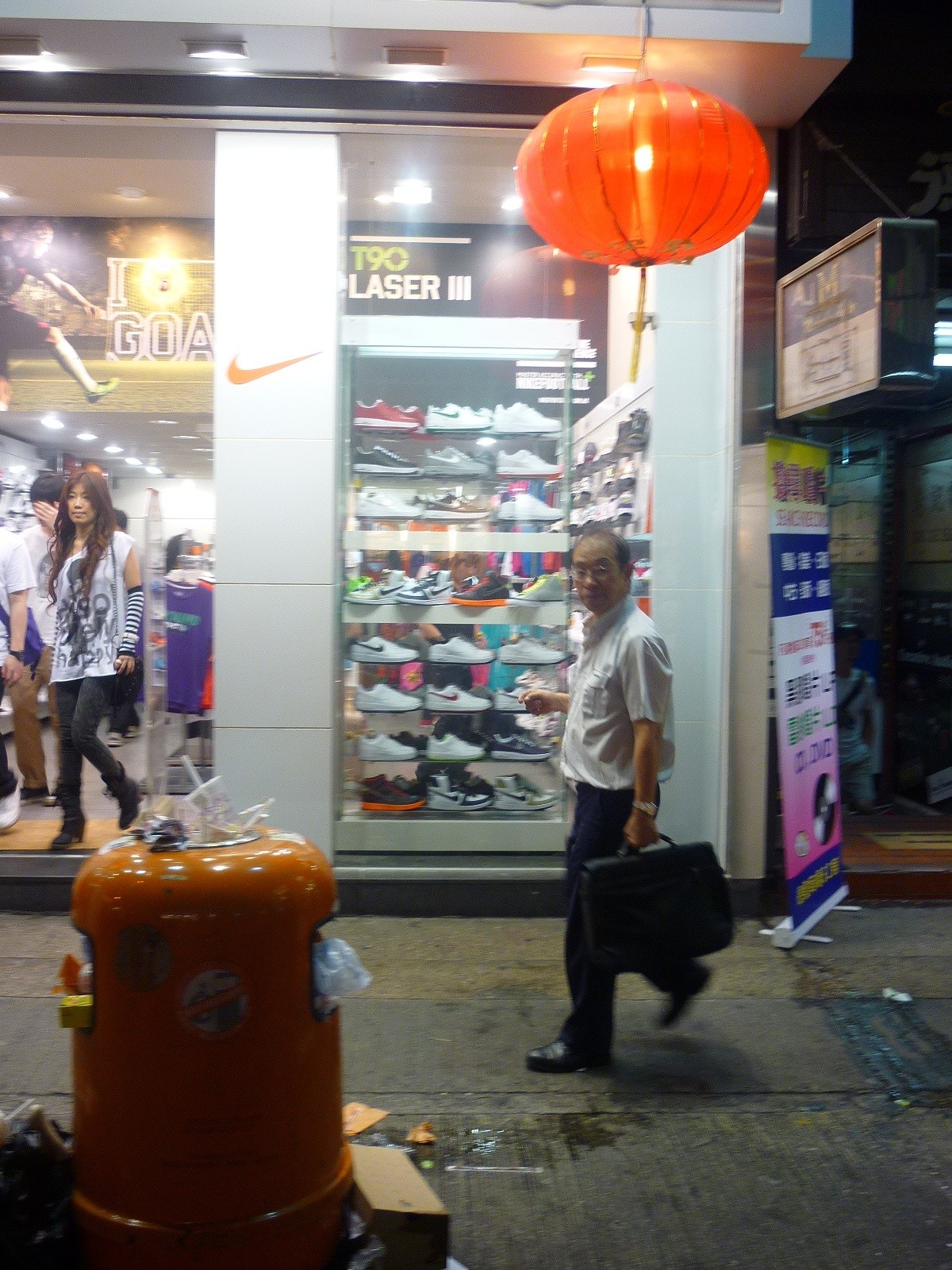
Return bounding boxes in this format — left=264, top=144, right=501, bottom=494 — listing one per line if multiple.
left=527, top=1040, right=612, bottom=1068
left=662, top=971, right=710, bottom=1026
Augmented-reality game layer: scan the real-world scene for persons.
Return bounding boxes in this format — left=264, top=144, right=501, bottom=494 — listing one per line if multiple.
left=517, top=530, right=709, bottom=1073
left=831, top=657, right=885, bottom=814
left=0, top=463, right=147, bottom=847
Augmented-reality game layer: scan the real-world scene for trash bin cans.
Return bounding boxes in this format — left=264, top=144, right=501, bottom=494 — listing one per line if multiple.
left=77, top=834, right=355, bottom=1270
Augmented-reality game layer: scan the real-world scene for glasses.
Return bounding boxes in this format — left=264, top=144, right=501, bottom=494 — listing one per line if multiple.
left=569, top=567, right=613, bottom=584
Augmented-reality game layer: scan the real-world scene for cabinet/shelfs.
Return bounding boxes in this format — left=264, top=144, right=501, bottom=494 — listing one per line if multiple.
left=336, top=344, right=575, bottom=853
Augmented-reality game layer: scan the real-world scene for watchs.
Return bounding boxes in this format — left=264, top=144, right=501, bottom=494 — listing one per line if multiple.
left=8, top=648, right=26, bottom=661
left=631, top=798, right=658, bottom=819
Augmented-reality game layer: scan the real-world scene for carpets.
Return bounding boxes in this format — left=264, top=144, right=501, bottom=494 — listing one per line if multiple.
left=0, top=818, right=131, bottom=850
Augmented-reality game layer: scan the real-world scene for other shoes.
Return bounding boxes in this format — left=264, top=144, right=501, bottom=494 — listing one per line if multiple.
left=20, top=787, right=49, bottom=805
left=126, top=726, right=140, bottom=737
left=42, top=788, right=60, bottom=805
left=107, top=732, right=123, bottom=745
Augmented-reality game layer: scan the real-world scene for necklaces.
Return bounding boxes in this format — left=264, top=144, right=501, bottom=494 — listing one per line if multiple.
left=70, top=535, right=81, bottom=617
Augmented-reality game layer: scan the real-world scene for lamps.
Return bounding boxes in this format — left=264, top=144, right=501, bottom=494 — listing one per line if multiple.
left=382, top=46, right=448, bottom=65
left=180, top=39, right=248, bottom=59
left=580, top=54, right=641, bottom=72
left=0, top=35, right=54, bottom=57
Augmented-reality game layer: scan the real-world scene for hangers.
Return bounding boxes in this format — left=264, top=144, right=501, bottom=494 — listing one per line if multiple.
left=165, top=554, right=215, bottom=586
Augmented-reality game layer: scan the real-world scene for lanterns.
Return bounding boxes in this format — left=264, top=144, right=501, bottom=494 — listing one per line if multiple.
left=514, top=76, right=769, bottom=385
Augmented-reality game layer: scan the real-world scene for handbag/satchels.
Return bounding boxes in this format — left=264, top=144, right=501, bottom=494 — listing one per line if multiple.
left=112, top=660, right=143, bottom=706
left=581, top=833, right=735, bottom=969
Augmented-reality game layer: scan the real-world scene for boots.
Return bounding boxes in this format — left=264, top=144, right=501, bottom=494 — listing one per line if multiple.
left=50, top=786, right=85, bottom=849
left=101, top=761, right=144, bottom=829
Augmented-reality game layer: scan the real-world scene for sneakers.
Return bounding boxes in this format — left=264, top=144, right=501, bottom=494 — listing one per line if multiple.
left=0, top=770, right=22, bottom=829
left=344, top=399, right=650, bottom=813
left=87, top=378, right=120, bottom=404
